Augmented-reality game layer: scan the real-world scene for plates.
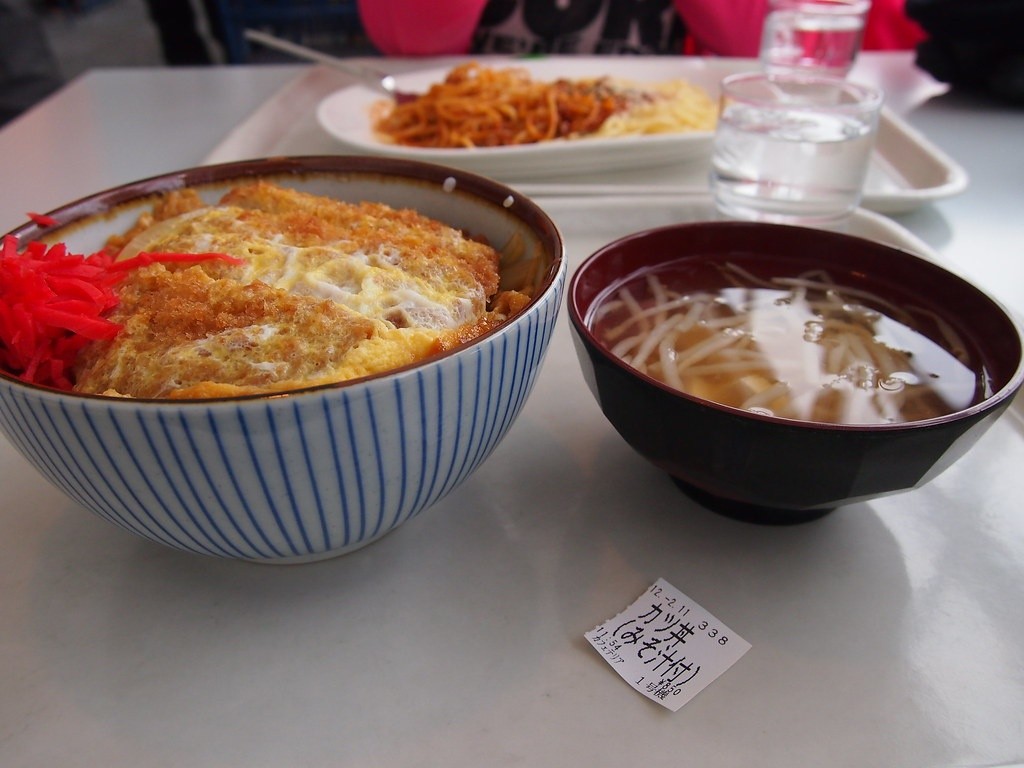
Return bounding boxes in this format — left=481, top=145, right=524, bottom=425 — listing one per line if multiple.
left=320, top=55, right=721, bottom=175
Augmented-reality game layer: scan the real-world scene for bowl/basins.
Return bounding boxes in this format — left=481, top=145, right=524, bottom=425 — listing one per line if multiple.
left=0, top=157, right=565, bottom=567
left=568, top=220, right=1023, bottom=526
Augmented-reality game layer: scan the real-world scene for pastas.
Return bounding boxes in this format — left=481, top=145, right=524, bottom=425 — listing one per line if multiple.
left=374, top=61, right=622, bottom=149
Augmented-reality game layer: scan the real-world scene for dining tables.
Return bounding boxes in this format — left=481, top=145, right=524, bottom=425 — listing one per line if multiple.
left=0, top=64, right=1022, bottom=766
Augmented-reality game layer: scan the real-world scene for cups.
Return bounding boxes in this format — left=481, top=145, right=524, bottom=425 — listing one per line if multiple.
left=762, top=0, right=872, bottom=81
left=711, top=71, right=883, bottom=225
